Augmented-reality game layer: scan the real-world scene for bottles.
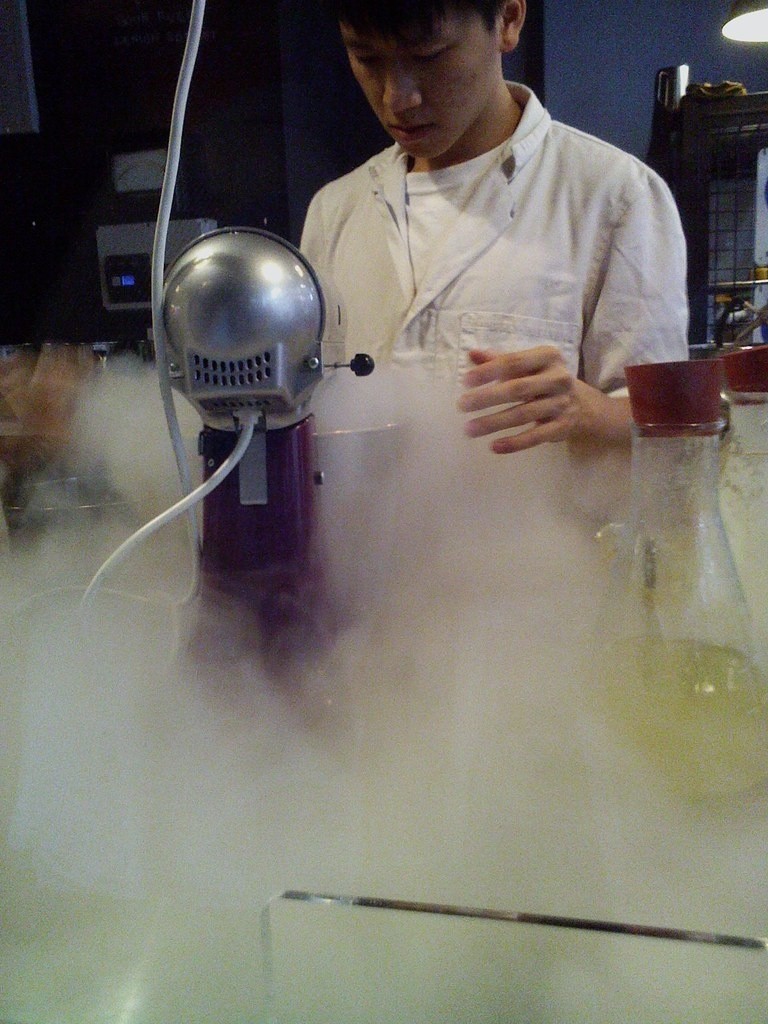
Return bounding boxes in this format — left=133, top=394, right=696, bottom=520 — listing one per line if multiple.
left=610, top=347, right=768, bottom=799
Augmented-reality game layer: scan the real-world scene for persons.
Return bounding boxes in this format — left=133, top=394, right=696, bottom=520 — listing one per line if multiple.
left=295, top=0, right=690, bottom=462
